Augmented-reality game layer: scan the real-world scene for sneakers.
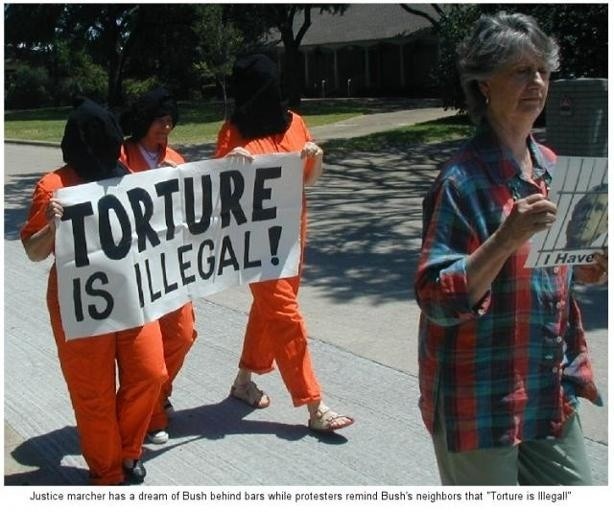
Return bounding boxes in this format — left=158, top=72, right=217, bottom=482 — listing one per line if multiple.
left=122, top=459, right=146, bottom=484
left=146, top=429, right=169, bottom=444
left=163, top=399, right=174, bottom=416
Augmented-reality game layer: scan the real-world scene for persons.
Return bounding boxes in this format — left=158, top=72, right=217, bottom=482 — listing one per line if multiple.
left=120, top=86, right=254, bottom=444
left=21, top=92, right=170, bottom=484
left=415, top=10, right=605, bottom=485
left=565, top=183, right=608, bottom=249
left=215, top=52, right=354, bottom=433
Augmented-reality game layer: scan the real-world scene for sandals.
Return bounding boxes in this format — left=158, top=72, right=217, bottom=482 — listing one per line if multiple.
left=309, top=407, right=354, bottom=432
left=231, top=380, right=269, bottom=408
